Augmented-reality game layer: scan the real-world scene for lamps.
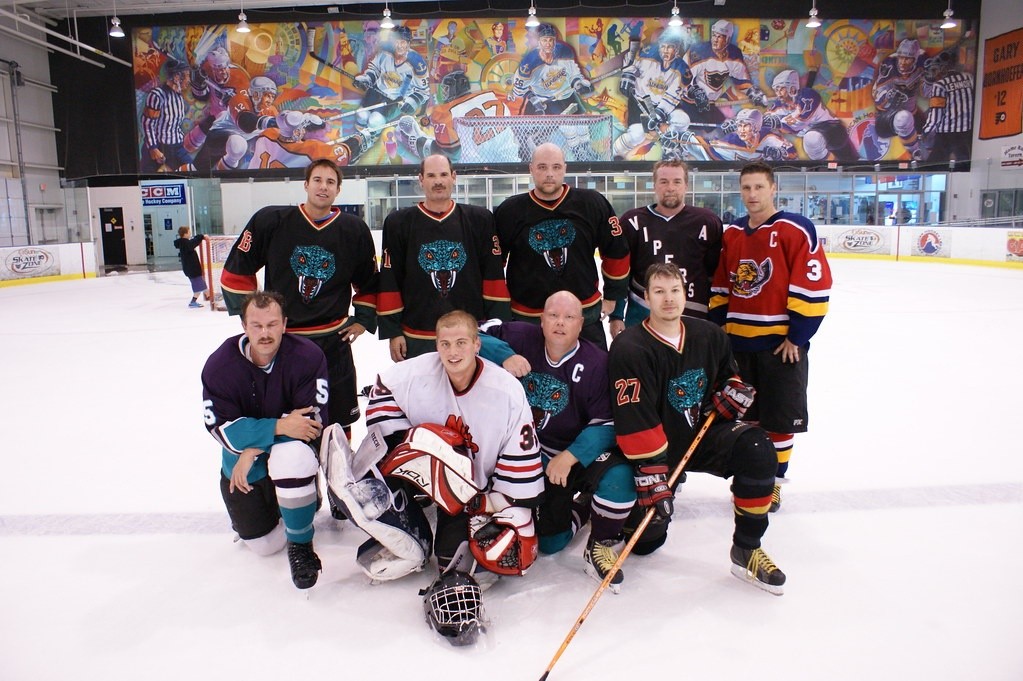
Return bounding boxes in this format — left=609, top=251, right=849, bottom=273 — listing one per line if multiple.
left=940, top=0, right=957, bottom=28
left=805, top=0, right=822, bottom=28
left=236, top=0, right=252, bottom=32
left=526, top=0, right=540, bottom=27
left=379, top=0, right=394, bottom=28
left=669, top=0, right=683, bottom=28
left=109, top=0, right=127, bottom=38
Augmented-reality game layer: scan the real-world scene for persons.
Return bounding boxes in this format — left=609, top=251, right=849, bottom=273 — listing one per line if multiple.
left=477, top=290, right=615, bottom=554
left=222, top=159, right=378, bottom=529
left=708, top=162, right=833, bottom=514
left=608, top=160, right=724, bottom=492
left=492, top=143, right=631, bottom=352
left=724, top=207, right=735, bottom=224
left=174, top=226, right=210, bottom=307
left=582, top=265, right=786, bottom=595
left=319, top=312, right=546, bottom=592
left=902, top=201, right=912, bottom=223
left=201, top=293, right=328, bottom=588
left=780, top=199, right=789, bottom=212
left=377, top=154, right=492, bottom=362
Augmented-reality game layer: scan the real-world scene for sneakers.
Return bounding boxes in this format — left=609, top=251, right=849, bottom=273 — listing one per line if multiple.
left=582, top=530, right=624, bottom=594
left=189, top=302, right=204, bottom=308
left=768, top=478, right=782, bottom=514
left=730, top=542, right=787, bottom=596
left=288, top=539, right=323, bottom=600
left=327, top=488, right=349, bottom=529
left=206, top=293, right=224, bottom=301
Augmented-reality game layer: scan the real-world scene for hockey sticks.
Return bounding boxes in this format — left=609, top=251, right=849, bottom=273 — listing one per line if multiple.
left=538, top=409, right=721, bottom=681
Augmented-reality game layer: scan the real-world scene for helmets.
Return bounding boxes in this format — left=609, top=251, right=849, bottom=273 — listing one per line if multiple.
left=420, top=569, right=483, bottom=639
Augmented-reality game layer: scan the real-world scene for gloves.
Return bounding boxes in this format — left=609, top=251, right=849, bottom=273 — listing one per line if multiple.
left=699, top=377, right=756, bottom=425
left=632, top=451, right=674, bottom=525
